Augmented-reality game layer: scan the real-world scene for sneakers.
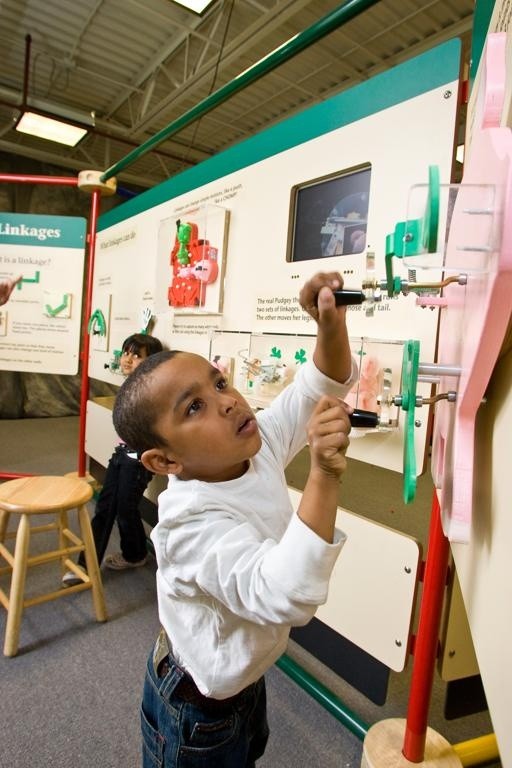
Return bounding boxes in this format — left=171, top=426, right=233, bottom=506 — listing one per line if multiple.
left=62, top=565, right=87, bottom=585
left=103, top=551, right=146, bottom=570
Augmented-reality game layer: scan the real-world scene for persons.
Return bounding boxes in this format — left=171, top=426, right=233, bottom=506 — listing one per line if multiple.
left=62, top=334, right=163, bottom=585
left=113, top=272, right=358, bottom=768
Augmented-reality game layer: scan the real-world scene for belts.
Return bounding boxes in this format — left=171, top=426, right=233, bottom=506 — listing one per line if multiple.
left=158, top=659, right=208, bottom=707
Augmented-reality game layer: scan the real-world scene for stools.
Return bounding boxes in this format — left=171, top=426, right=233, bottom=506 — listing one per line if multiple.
left=2, top=474, right=108, bottom=656
